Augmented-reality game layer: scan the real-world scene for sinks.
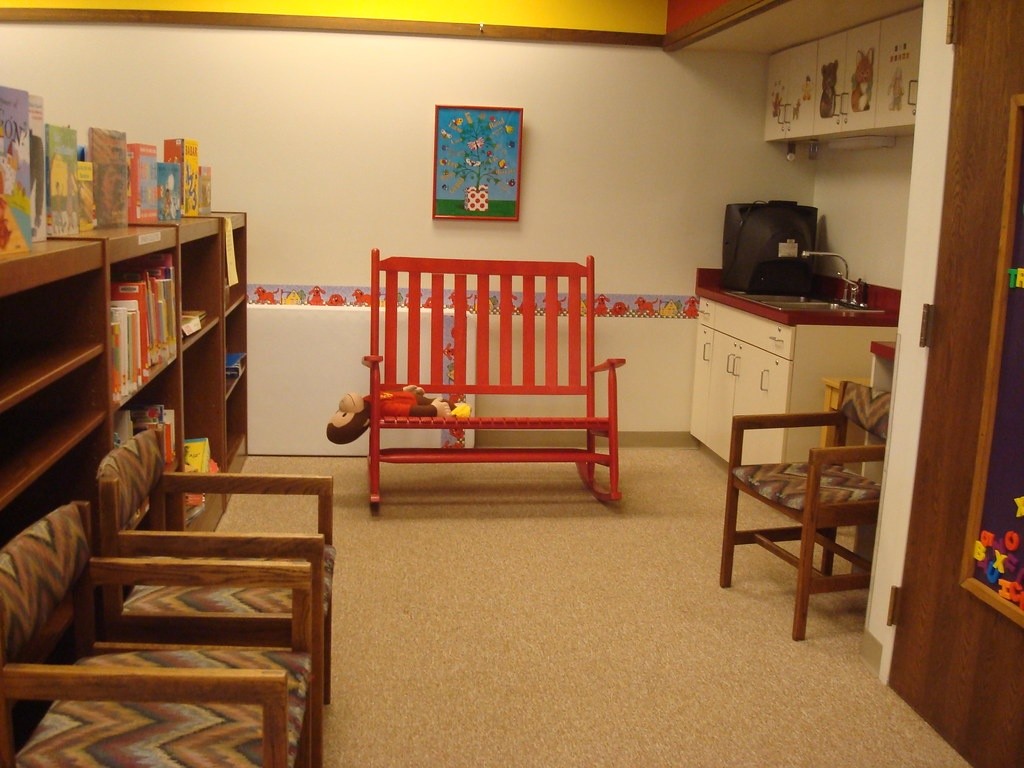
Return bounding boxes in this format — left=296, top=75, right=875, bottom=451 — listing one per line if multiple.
left=759, top=299, right=849, bottom=311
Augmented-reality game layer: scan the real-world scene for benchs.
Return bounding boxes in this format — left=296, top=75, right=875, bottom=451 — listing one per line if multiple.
left=360, top=247, right=626, bottom=518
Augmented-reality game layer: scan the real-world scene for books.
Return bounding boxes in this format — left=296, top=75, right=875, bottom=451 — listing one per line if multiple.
left=182, top=307, right=209, bottom=338
left=110, top=253, right=178, bottom=403
left=114, top=405, right=221, bottom=525
left=0, top=86, right=212, bottom=248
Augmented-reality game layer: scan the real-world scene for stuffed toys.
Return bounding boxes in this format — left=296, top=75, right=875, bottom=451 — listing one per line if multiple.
left=327, top=384, right=472, bottom=444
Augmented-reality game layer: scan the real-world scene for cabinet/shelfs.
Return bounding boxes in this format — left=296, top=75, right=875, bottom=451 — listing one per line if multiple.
left=861, top=340, right=896, bottom=484
left=813, top=20, right=880, bottom=136
left=0, top=211, right=248, bottom=533
left=819, top=379, right=870, bottom=468
left=690, top=296, right=899, bottom=464
left=874, top=7, right=923, bottom=135
left=764, top=40, right=818, bottom=141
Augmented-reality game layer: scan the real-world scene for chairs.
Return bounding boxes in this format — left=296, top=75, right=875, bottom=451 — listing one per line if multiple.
left=97, top=424, right=334, bottom=705
left=0, top=502, right=325, bottom=768
left=718, top=381, right=892, bottom=640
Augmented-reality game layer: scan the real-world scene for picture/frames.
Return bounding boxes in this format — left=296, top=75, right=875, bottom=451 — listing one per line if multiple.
left=432, top=105, right=523, bottom=222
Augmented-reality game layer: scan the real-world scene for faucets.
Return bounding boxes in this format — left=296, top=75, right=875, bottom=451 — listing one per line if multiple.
left=802, top=250, right=858, bottom=306
